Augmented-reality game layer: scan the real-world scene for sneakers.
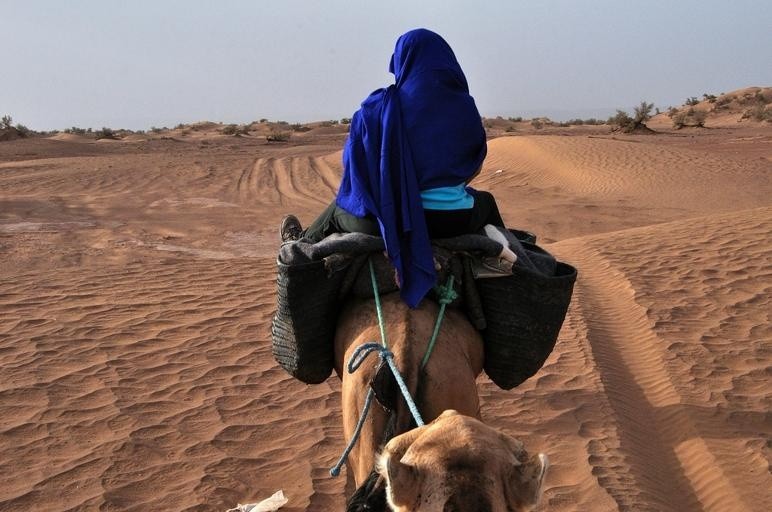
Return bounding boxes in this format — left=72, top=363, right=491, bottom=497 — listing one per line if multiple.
left=279, top=215, right=303, bottom=243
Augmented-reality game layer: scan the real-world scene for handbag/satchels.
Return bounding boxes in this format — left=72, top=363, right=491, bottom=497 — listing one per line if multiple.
left=271, top=247, right=360, bottom=384
left=467, top=228, right=577, bottom=390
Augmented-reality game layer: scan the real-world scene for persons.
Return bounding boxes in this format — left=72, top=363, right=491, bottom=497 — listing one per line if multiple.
left=278, top=28, right=506, bottom=239
